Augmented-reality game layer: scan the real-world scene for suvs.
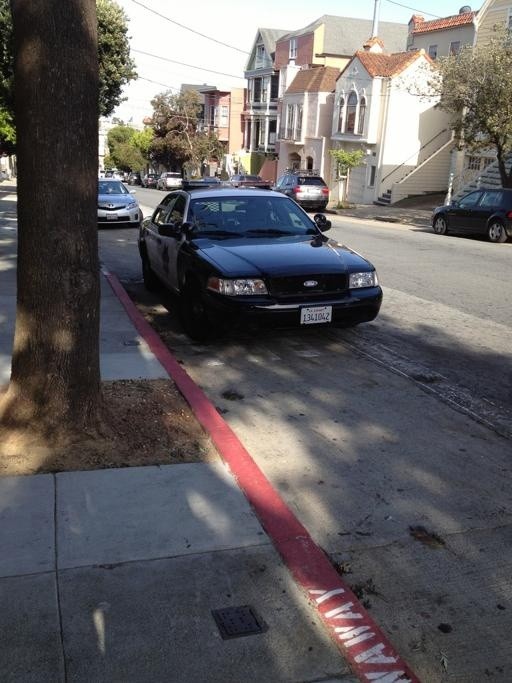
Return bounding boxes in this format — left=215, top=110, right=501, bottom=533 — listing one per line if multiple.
left=275, top=175, right=330, bottom=210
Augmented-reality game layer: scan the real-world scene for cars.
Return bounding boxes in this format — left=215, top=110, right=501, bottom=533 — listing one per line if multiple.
left=138, top=186, right=384, bottom=341
left=430, top=188, right=512, bottom=243
left=102, top=170, right=181, bottom=190
left=227, top=175, right=270, bottom=188
left=97, top=178, right=143, bottom=227
